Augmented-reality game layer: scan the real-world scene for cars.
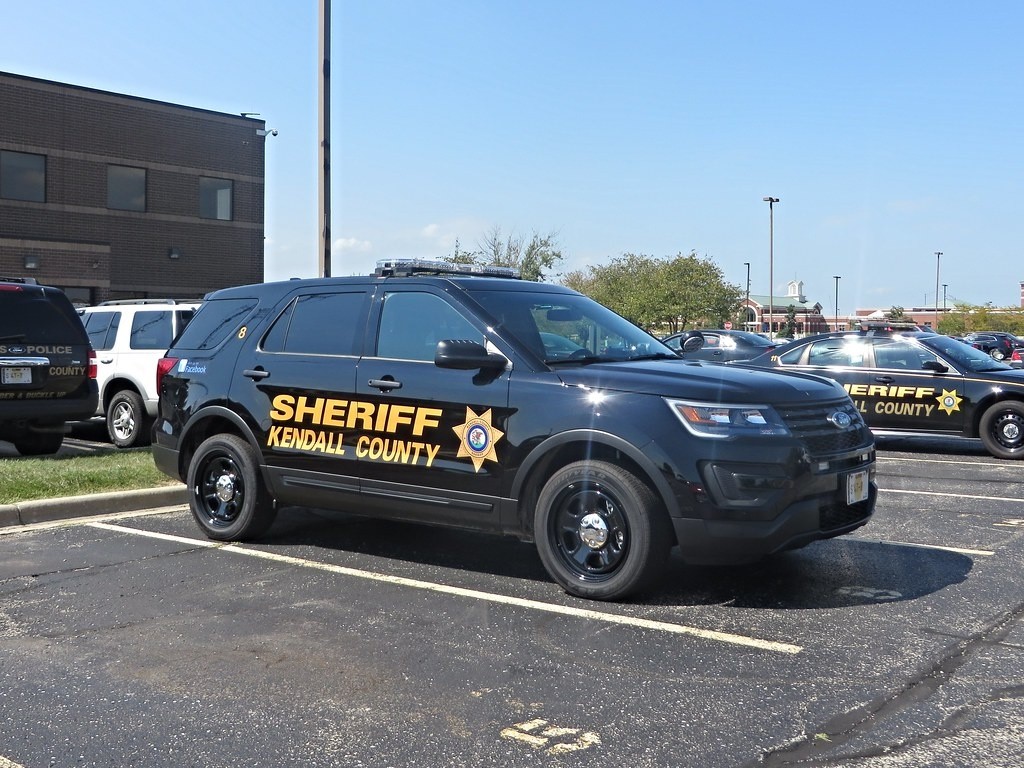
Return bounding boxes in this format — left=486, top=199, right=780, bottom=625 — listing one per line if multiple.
left=727, top=320, right=1024, bottom=460
left=908, top=323, right=1024, bottom=370
left=604, top=328, right=796, bottom=362
left=536, top=327, right=593, bottom=362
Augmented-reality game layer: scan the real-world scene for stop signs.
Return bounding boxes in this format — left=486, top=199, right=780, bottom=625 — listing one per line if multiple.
left=724, top=321, right=732, bottom=330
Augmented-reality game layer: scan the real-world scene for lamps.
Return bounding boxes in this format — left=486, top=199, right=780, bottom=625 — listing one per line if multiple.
left=169, top=248, right=180, bottom=258
left=24, top=256, right=39, bottom=269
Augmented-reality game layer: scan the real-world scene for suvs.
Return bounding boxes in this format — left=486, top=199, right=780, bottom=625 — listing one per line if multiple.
left=148, top=258, right=880, bottom=604
left=0, top=275, right=101, bottom=457
left=67, top=298, right=204, bottom=449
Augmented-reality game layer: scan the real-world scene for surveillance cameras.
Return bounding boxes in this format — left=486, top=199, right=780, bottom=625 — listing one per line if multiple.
left=272, top=130, right=278, bottom=136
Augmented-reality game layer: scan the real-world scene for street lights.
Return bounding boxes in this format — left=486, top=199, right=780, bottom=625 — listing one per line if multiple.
left=942, top=284, right=947, bottom=314
left=744, top=262, right=751, bottom=333
left=762, top=195, right=781, bottom=339
left=833, top=275, right=842, bottom=332
left=934, top=251, right=944, bottom=334
